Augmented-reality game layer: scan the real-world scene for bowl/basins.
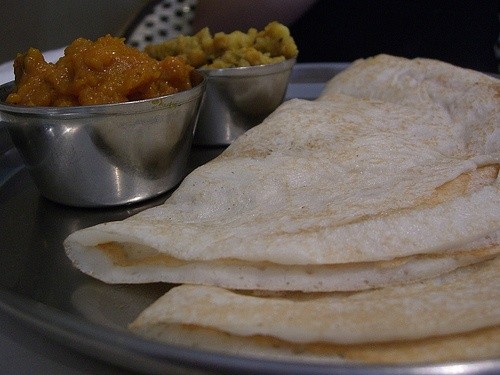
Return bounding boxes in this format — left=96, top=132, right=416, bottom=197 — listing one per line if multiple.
left=198, top=59, right=296, bottom=144
left=1, top=69, right=207, bottom=205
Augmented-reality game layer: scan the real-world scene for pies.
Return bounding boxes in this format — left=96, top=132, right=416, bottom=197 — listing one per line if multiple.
left=63, top=54, right=500, bottom=362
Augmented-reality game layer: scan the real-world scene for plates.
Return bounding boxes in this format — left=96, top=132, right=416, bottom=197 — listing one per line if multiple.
left=0, top=63, right=500, bottom=375
